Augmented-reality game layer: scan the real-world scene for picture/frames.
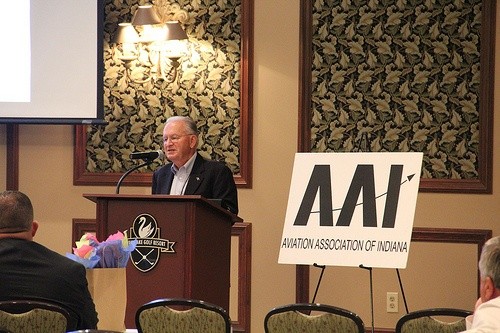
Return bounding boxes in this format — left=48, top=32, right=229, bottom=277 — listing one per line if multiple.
left=295, top=225, right=492, bottom=333
left=298, top=0, right=496, bottom=194
left=69, top=0, right=254, bottom=188
left=72, top=218, right=252, bottom=333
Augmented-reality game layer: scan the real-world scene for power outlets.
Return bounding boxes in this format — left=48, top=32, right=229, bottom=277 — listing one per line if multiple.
left=386, top=293, right=398, bottom=313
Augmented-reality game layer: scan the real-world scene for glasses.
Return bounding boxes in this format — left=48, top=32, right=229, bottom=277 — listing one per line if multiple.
left=163, top=133, right=194, bottom=142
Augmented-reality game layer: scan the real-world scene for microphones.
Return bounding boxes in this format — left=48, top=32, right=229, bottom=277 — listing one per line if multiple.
left=128, top=150, right=164, bottom=159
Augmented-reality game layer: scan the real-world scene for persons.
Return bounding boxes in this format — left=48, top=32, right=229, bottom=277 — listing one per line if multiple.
left=457, top=236, right=500, bottom=333
left=0, top=190, right=99, bottom=333
left=152, top=116, right=238, bottom=227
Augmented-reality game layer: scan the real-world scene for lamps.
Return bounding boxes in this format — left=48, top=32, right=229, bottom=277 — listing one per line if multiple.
left=116, top=6, right=189, bottom=83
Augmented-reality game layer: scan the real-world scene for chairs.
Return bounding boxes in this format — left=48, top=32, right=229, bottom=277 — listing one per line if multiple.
left=395, top=308, right=473, bottom=333
left=0, top=294, right=80, bottom=333
left=135, top=298, right=231, bottom=333
left=263, top=303, right=365, bottom=333
